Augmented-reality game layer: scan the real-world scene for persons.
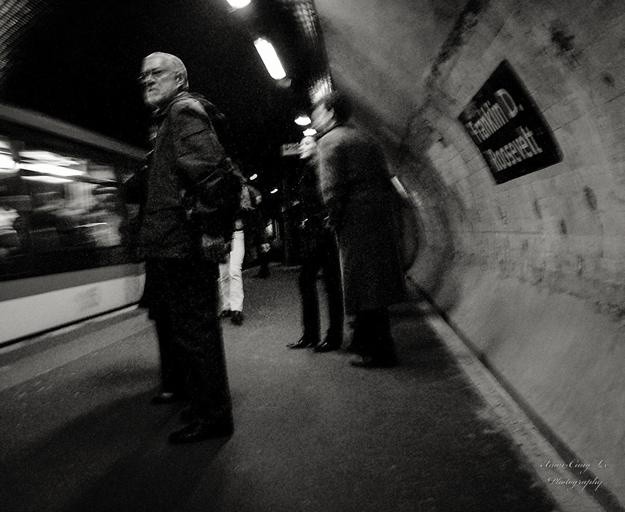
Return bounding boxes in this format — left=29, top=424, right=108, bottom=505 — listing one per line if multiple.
left=249, top=192, right=277, bottom=279
left=286, top=89, right=408, bottom=373
left=135, top=52, right=242, bottom=440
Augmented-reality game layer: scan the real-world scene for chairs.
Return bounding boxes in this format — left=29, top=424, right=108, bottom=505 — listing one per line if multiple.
left=151, top=390, right=180, bottom=406
left=286, top=335, right=321, bottom=349
left=219, top=310, right=232, bottom=319
left=314, top=339, right=342, bottom=353
left=169, top=420, right=233, bottom=444
left=230, top=311, right=241, bottom=322
left=347, top=344, right=401, bottom=368
